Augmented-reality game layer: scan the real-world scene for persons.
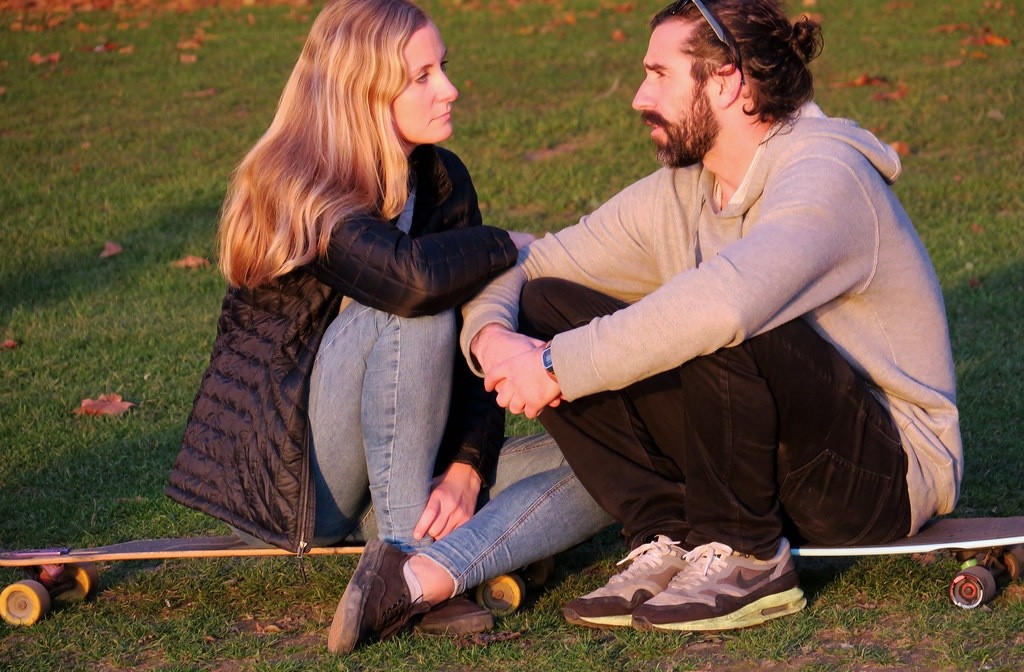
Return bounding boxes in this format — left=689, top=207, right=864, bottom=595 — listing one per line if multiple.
left=460, top=0, right=963, bottom=630
left=158, top=0, right=616, bottom=654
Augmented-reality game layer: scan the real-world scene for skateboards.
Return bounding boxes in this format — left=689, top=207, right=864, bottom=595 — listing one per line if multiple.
left=0, top=529, right=543, bottom=627
left=617, top=512, right=1024, bottom=613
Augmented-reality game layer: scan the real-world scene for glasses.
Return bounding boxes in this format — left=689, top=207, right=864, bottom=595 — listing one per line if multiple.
left=674, top=0, right=745, bottom=86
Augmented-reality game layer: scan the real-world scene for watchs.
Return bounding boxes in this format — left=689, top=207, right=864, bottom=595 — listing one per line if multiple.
left=541, top=340, right=558, bottom=383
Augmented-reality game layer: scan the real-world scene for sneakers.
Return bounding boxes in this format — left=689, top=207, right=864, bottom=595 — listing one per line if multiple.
left=328, top=539, right=430, bottom=655
left=414, top=594, right=493, bottom=634
left=631, top=538, right=807, bottom=632
left=560, top=533, right=691, bottom=628
